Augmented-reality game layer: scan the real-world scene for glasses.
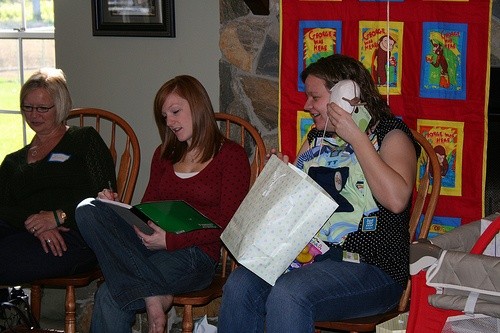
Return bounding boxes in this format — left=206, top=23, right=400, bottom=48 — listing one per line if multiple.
left=21, top=100, right=55, bottom=113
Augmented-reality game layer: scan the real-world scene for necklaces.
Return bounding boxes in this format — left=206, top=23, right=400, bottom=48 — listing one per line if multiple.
left=29, top=146, right=43, bottom=159
left=189, top=157, right=195, bottom=164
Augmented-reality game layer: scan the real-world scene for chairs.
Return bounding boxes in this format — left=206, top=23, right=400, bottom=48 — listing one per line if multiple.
left=14, top=108, right=141, bottom=333
left=314, top=127, right=442, bottom=333
left=151, top=113, right=267, bottom=333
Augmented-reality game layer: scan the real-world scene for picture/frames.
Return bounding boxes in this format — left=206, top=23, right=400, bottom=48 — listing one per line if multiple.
left=90, top=0, right=177, bottom=39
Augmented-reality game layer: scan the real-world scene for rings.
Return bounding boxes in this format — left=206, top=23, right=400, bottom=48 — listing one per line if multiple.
left=46, top=238, right=52, bottom=244
left=32, top=225, right=39, bottom=233
left=142, top=238, right=144, bottom=244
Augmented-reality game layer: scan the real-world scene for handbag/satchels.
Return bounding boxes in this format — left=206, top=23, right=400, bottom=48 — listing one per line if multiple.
left=220, top=154, right=339, bottom=286
left=0, top=295, right=40, bottom=331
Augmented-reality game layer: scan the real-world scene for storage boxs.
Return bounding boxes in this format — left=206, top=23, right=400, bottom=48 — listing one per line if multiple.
left=405, top=210, right=500, bottom=333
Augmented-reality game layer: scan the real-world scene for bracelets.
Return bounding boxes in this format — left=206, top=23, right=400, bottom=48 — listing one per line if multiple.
left=57, top=208, right=67, bottom=222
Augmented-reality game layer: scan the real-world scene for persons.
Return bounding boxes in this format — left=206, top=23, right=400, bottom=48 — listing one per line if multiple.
left=1, top=66, right=119, bottom=333
left=220, top=54, right=420, bottom=333
left=74, top=74, right=253, bottom=333
